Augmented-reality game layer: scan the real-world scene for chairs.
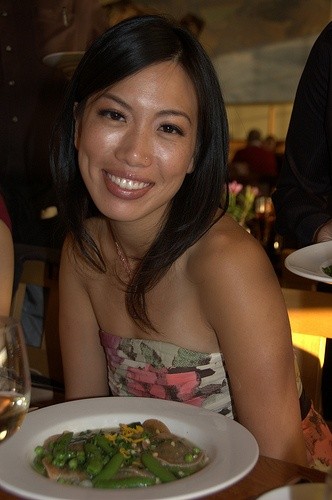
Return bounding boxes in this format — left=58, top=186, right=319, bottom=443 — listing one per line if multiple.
left=14, top=244, right=63, bottom=390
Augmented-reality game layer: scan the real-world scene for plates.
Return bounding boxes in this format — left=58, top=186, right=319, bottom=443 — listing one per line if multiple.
left=284, top=241, right=332, bottom=285
left=0, top=396, right=259, bottom=500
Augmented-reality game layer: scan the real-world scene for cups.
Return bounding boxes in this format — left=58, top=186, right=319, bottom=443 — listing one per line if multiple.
left=0, top=314, right=31, bottom=443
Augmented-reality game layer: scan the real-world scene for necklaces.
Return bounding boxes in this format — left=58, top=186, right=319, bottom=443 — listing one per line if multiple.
left=112, top=234, right=134, bottom=282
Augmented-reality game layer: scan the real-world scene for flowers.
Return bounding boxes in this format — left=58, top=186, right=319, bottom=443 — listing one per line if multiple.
left=221, top=180, right=258, bottom=234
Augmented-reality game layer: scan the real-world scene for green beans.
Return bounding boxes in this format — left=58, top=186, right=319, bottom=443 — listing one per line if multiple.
left=33, top=430, right=200, bottom=488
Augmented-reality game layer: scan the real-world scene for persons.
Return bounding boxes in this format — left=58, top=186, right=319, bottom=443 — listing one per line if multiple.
left=226, top=129, right=286, bottom=196
left=49, top=13, right=332, bottom=476
left=280, top=21, right=332, bottom=247
left=0, top=0, right=140, bottom=347
